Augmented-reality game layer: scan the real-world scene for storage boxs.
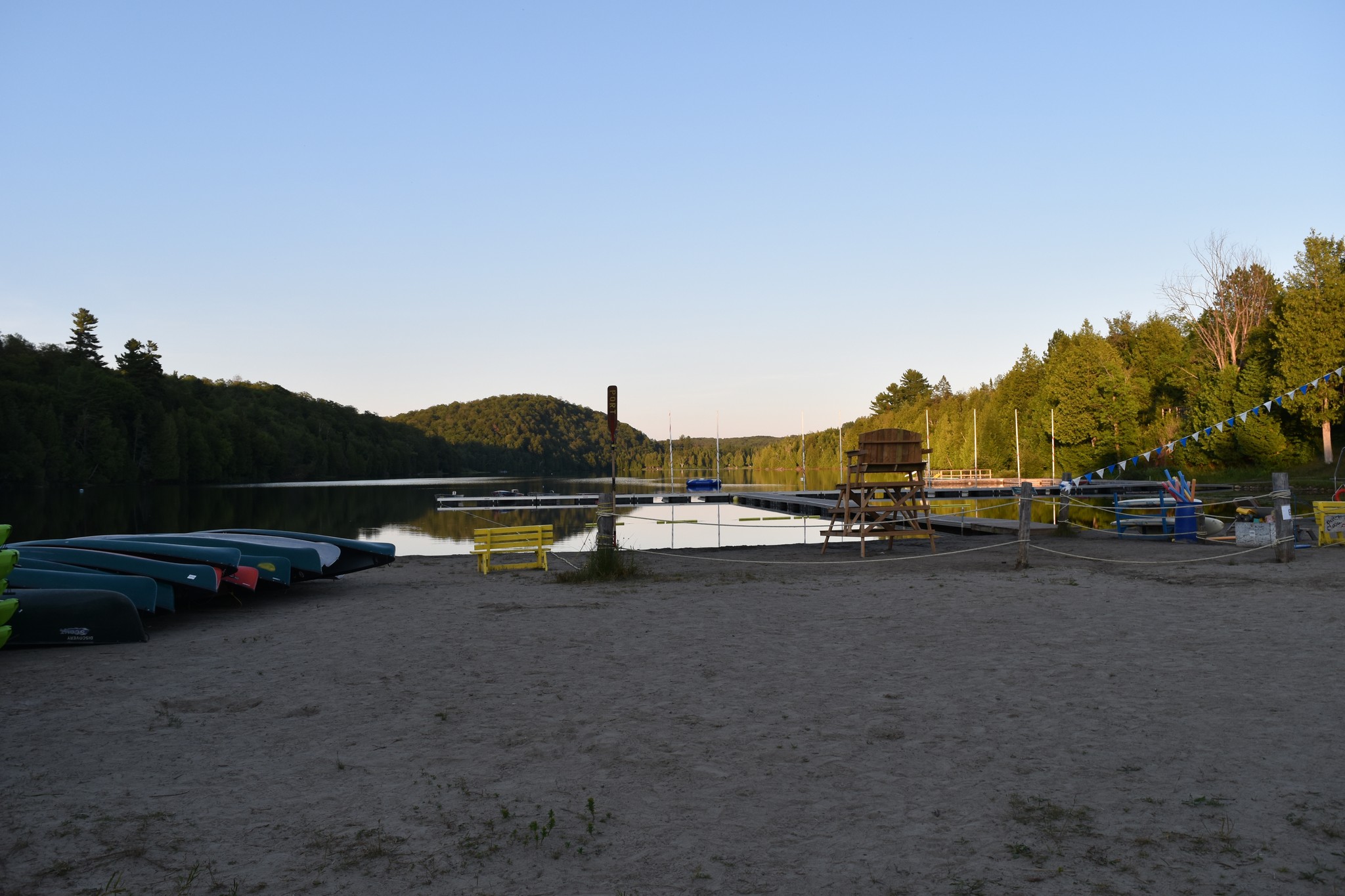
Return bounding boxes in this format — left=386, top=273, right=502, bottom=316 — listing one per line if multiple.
left=1235, top=506, right=1276, bottom=548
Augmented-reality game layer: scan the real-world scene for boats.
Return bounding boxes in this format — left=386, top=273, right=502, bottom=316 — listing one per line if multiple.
left=1110, top=516, right=1224, bottom=534
left=526, top=490, right=561, bottom=501
left=0, top=522, right=398, bottom=650
left=435, top=491, right=464, bottom=501
left=1112, top=497, right=1203, bottom=508
left=686, top=478, right=722, bottom=487
left=491, top=489, right=524, bottom=502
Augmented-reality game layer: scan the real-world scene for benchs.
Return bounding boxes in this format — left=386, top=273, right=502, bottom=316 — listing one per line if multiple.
left=470, top=525, right=553, bottom=575
left=1313, top=501, right=1345, bottom=547
left=1293, top=517, right=1318, bottom=542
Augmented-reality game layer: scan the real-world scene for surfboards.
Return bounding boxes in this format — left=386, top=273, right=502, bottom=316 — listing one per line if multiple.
left=1110, top=497, right=1225, bottom=536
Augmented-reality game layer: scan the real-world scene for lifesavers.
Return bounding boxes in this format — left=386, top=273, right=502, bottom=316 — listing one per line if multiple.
left=1335, top=488, right=1345, bottom=502
left=1162, top=470, right=1196, bottom=503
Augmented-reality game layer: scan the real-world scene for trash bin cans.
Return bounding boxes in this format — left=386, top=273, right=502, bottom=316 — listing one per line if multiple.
left=1174, top=502, right=1205, bottom=543
